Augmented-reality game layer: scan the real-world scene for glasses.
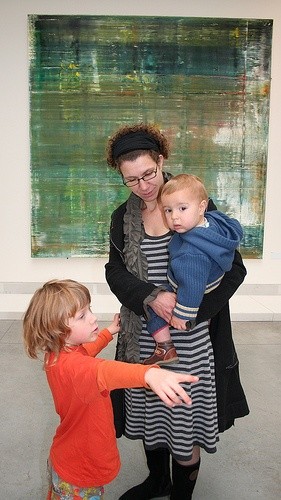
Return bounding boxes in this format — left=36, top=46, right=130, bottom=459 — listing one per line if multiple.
left=123, top=158, right=157, bottom=187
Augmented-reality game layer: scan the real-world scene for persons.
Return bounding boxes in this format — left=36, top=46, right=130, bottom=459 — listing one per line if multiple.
left=142, top=173, right=242, bottom=367
left=23, top=278, right=199, bottom=500
left=102, top=122, right=250, bottom=500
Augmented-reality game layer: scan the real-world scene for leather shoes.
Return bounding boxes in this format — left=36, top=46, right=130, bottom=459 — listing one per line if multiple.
left=144, top=341, right=181, bottom=368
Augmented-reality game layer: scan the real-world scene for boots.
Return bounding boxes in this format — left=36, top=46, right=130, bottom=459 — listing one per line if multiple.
left=118, top=444, right=173, bottom=500
left=172, top=458, right=202, bottom=500
